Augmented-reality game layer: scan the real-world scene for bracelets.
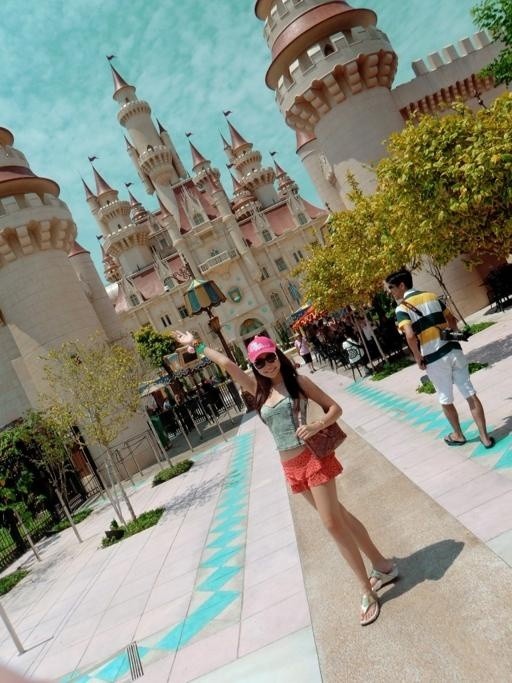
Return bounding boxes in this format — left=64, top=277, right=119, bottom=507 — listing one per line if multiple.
left=187, top=341, right=204, bottom=355
left=318, top=420, right=327, bottom=431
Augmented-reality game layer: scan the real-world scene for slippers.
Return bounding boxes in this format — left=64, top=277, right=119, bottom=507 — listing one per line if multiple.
left=443, top=431, right=466, bottom=446
left=359, top=587, right=381, bottom=626
left=480, top=434, right=496, bottom=449
left=367, top=558, right=400, bottom=592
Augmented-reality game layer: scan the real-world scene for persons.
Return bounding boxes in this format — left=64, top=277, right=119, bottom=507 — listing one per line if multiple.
left=0, top=497, right=27, bottom=554
left=145, top=370, right=233, bottom=434
left=168, top=329, right=399, bottom=626
left=385, top=270, right=495, bottom=448
left=477, top=257, right=508, bottom=308
left=294, top=291, right=405, bottom=375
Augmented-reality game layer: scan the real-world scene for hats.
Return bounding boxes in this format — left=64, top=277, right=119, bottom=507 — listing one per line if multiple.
left=247, top=335, right=277, bottom=364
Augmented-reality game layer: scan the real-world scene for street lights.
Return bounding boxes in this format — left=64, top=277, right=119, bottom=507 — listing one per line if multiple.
left=162, top=253, right=258, bottom=414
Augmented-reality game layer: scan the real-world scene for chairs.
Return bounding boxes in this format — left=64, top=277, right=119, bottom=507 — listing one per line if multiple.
left=311, top=336, right=406, bottom=383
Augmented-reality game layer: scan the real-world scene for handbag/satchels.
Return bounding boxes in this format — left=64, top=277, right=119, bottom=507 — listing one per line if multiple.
left=304, top=421, right=347, bottom=460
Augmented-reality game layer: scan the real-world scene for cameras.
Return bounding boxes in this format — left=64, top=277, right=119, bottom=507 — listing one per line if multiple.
left=440, top=327, right=469, bottom=341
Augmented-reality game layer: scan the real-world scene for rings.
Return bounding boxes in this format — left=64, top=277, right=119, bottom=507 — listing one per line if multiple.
left=179, top=334, right=182, bottom=338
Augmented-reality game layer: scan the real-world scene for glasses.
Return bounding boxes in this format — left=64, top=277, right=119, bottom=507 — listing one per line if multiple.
left=387, top=284, right=402, bottom=289
left=253, top=353, right=277, bottom=370
